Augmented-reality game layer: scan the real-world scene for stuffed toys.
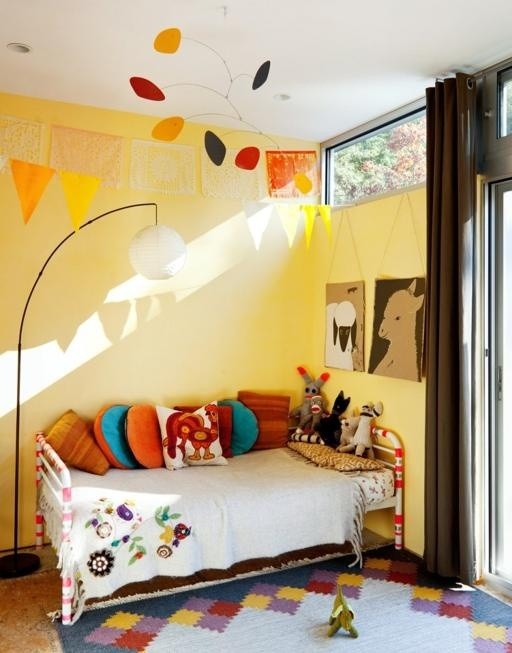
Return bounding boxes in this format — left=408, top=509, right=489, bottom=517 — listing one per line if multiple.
left=287, top=363, right=384, bottom=459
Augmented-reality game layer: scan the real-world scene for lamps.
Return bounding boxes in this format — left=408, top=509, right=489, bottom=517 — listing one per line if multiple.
left=2, top=202, right=187, bottom=580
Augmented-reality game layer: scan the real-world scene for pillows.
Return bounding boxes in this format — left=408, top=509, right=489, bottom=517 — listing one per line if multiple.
left=44, top=390, right=292, bottom=475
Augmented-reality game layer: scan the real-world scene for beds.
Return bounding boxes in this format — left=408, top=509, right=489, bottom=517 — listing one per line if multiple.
left=35, top=411, right=405, bottom=626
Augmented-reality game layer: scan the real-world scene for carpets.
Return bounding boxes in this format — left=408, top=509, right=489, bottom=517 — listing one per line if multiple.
left=46, top=538, right=510, bottom=650
